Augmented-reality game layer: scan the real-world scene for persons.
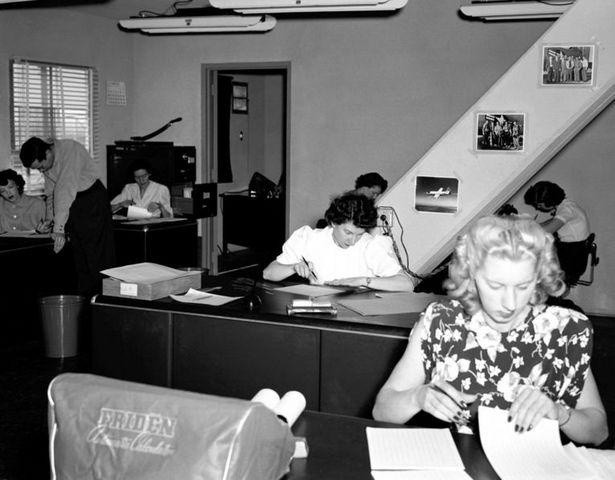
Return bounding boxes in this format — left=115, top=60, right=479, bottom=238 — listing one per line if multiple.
left=523, top=180, right=589, bottom=242
left=482, top=117, right=520, bottom=151
left=372, top=212, right=610, bottom=446
left=20, top=136, right=114, bottom=297
left=110, top=162, right=175, bottom=218
left=262, top=194, right=416, bottom=293
left=0, top=169, right=49, bottom=233
left=549, top=50, right=588, bottom=83
left=344, top=171, right=389, bottom=199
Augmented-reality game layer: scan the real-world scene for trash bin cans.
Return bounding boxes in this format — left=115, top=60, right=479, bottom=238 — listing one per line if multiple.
left=39, top=296, right=83, bottom=358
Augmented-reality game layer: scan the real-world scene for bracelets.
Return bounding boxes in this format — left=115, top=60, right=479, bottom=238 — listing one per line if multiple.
left=557, top=399, right=572, bottom=427
left=366, top=277, right=370, bottom=290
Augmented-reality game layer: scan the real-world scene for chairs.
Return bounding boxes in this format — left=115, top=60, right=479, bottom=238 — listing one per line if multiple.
left=563, top=233, right=599, bottom=286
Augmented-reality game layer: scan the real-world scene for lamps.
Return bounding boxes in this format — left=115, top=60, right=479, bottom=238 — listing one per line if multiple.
left=459, top=1, right=575, bottom=22
left=118, top=14, right=277, bottom=37
left=208, top=0, right=408, bottom=16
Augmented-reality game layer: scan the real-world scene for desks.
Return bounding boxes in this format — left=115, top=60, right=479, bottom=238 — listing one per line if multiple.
left=0, top=236, right=73, bottom=369
left=114, top=217, right=199, bottom=270
left=277, top=409, right=614, bottom=480
left=218, top=192, right=286, bottom=271
left=89, top=275, right=453, bottom=428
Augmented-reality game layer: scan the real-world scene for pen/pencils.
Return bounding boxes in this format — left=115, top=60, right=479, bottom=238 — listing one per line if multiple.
left=302, top=255, right=317, bottom=280
left=131, top=198, right=133, bottom=205
left=41, top=216, right=44, bottom=225
left=535, top=214, right=538, bottom=220
left=433, top=384, right=465, bottom=410
